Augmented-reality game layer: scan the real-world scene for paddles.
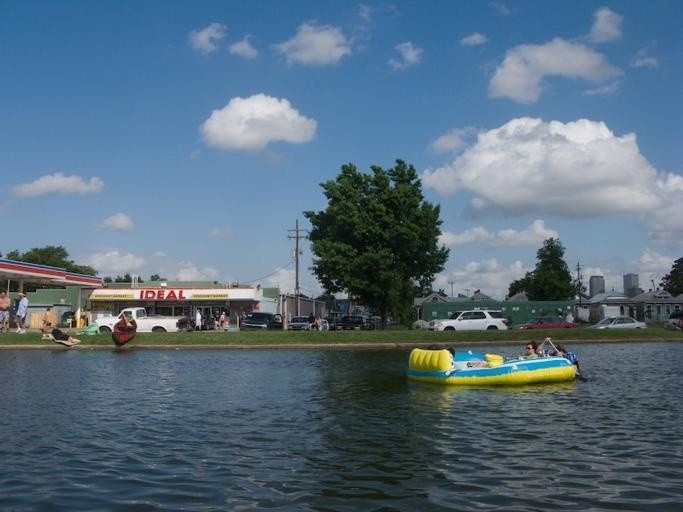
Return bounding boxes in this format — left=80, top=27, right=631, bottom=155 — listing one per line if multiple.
left=120, top=310, right=132, bottom=327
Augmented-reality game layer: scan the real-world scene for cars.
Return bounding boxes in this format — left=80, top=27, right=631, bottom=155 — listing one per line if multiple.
left=512, top=316, right=580, bottom=330
left=586, top=316, right=648, bottom=330
left=427, top=309, right=509, bottom=331
left=188, top=314, right=215, bottom=330
left=240, top=312, right=382, bottom=330
left=61, top=311, right=76, bottom=327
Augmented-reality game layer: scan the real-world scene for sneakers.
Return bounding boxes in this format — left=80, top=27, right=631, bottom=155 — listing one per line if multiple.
left=0, top=326, right=27, bottom=335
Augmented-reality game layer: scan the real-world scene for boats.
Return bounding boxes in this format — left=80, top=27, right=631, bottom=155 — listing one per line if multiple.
left=406, top=347, right=578, bottom=385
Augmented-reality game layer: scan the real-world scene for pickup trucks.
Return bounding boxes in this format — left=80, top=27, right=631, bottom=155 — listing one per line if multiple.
left=96, top=306, right=189, bottom=333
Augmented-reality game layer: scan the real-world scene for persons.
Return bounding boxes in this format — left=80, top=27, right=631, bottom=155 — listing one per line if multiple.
left=307, top=312, right=322, bottom=331
left=80, top=308, right=85, bottom=328
left=196, top=309, right=230, bottom=332
left=0, top=290, right=10, bottom=333
left=13, top=290, right=29, bottom=335
left=523, top=337, right=567, bottom=360
left=427, top=344, right=488, bottom=369
left=39, top=326, right=80, bottom=345
left=42, top=307, right=51, bottom=326
